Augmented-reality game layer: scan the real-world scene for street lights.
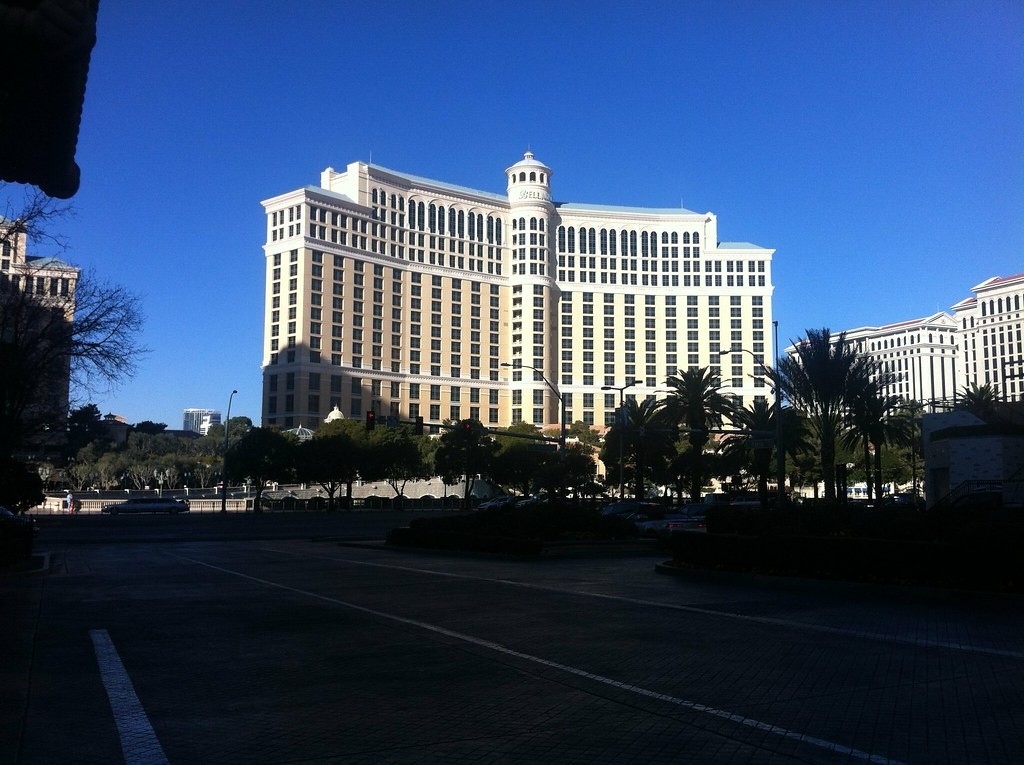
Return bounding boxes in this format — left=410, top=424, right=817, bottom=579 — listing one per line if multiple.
left=499, top=362, right=567, bottom=502
left=600, top=380, right=643, bottom=500
left=718, top=320, right=786, bottom=496
left=219, top=390, right=238, bottom=515
left=889, top=399, right=941, bottom=495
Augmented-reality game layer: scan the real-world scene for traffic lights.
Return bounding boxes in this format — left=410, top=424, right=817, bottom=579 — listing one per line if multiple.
left=462, top=422, right=480, bottom=441
left=415, top=415, right=423, bottom=435
left=365, top=410, right=376, bottom=430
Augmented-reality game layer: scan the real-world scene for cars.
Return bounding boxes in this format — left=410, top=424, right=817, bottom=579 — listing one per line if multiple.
left=477, top=487, right=925, bottom=538
left=0, top=505, right=44, bottom=547
left=101, top=494, right=188, bottom=515
